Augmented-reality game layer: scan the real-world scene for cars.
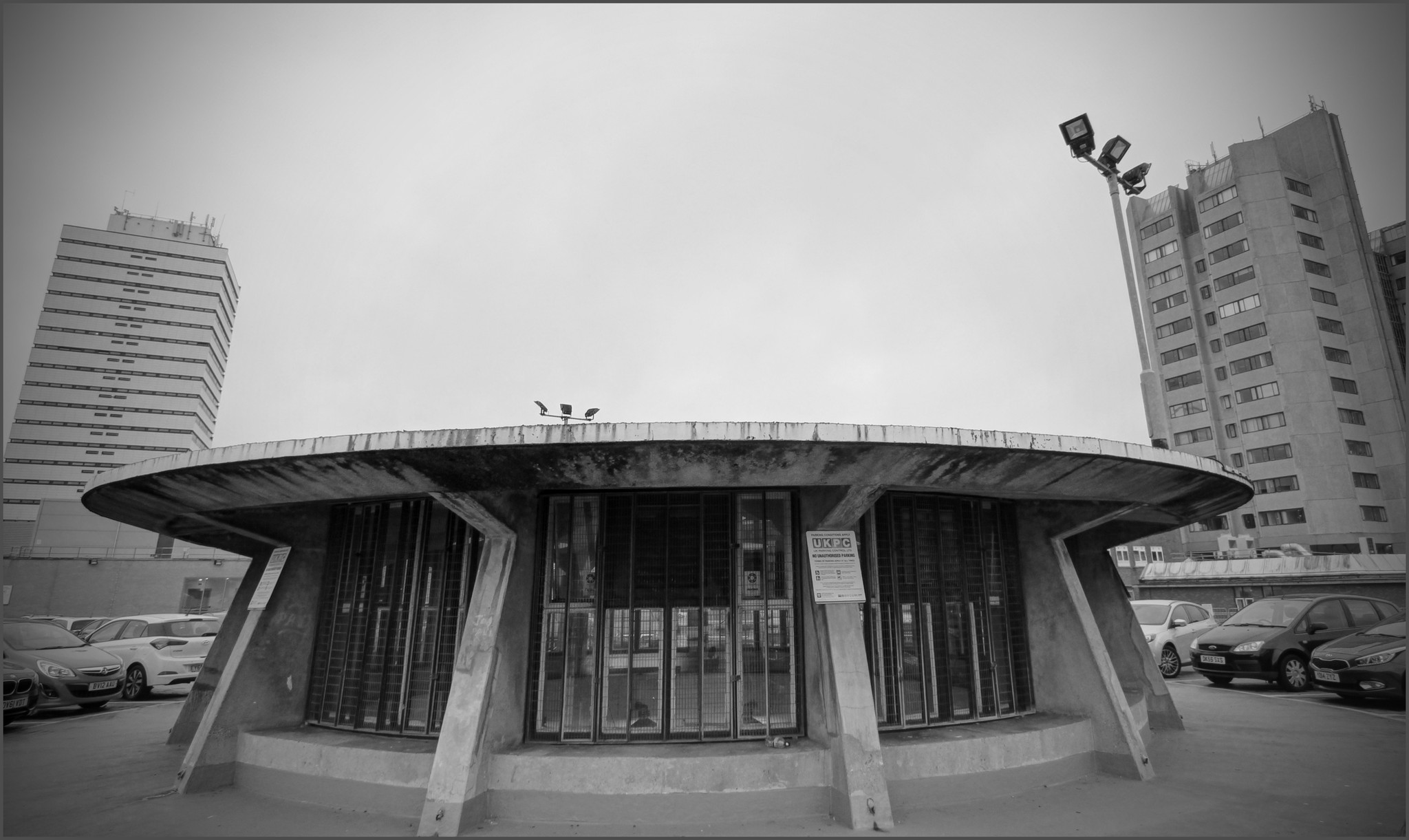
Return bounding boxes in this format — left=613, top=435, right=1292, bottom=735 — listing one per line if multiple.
left=21, top=612, right=228, bottom=702
left=3, top=617, right=127, bottom=717
left=1188, top=592, right=1403, bottom=693
left=1307, top=610, right=1406, bottom=711
left=3, top=659, right=41, bottom=728
left=1129, top=599, right=1218, bottom=678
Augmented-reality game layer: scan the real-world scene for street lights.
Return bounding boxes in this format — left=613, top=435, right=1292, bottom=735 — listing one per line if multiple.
left=536, top=399, right=600, bottom=426
left=1058, top=112, right=1171, bottom=451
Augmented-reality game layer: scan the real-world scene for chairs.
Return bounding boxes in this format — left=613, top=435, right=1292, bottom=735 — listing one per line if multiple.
left=1283, top=607, right=1298, bottom=625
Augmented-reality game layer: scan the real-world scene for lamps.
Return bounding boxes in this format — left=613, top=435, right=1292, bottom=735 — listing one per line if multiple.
left=538, top=401, right=599, bottom=424
left=214, top=560, right=222, bottom=565
left=89, top=559, right=98, bottom=565
